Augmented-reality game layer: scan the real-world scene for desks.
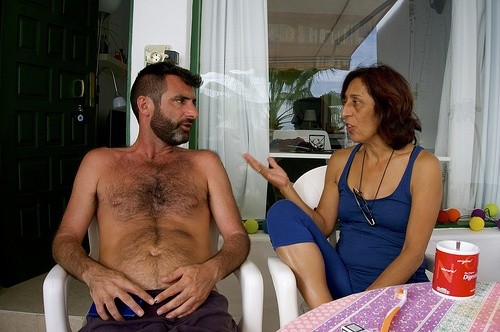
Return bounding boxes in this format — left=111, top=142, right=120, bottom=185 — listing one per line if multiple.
left=273, top=281, right=500, bottom=332
left=269, top=151, right=455, bottom=195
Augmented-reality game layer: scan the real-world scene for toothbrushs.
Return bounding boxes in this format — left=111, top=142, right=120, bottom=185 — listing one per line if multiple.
left=380, top=288, right=408, bottom=332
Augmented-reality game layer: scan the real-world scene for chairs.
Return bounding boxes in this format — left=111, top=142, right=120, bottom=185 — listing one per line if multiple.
left=43, top=215, right=264, bottom=332
left=267, top=164, right=436, bottom=328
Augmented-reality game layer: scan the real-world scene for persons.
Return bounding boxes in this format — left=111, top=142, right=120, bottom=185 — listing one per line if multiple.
left=243, top=63, right=444, bottom=312
left=291, top=97, right=343, bottom=178
left=51, top=61, right=251, bottom=332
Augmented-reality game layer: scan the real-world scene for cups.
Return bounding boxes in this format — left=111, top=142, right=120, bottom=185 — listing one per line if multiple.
left=429, top=240, right=480, bottom=300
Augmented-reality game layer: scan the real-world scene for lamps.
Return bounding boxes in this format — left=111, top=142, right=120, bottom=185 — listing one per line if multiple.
left=94, top=66, right=127, bottom=109
left=303, top=110, right=316, bottom=128
left=98, top=0, right=123, bottom=79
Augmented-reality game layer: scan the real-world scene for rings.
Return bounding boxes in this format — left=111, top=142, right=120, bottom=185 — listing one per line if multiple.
left=257, top=168, right=262, bottom=173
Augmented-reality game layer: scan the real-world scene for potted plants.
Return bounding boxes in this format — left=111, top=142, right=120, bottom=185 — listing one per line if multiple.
left=269, top=69, right=316, bottom=142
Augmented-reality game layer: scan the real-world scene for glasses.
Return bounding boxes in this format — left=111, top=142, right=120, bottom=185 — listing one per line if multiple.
left=351, top=187, right=377, bottom=228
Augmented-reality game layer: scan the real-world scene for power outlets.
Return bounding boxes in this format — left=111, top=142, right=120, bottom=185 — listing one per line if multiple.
left=145, top=45, right=170, bottom=66
